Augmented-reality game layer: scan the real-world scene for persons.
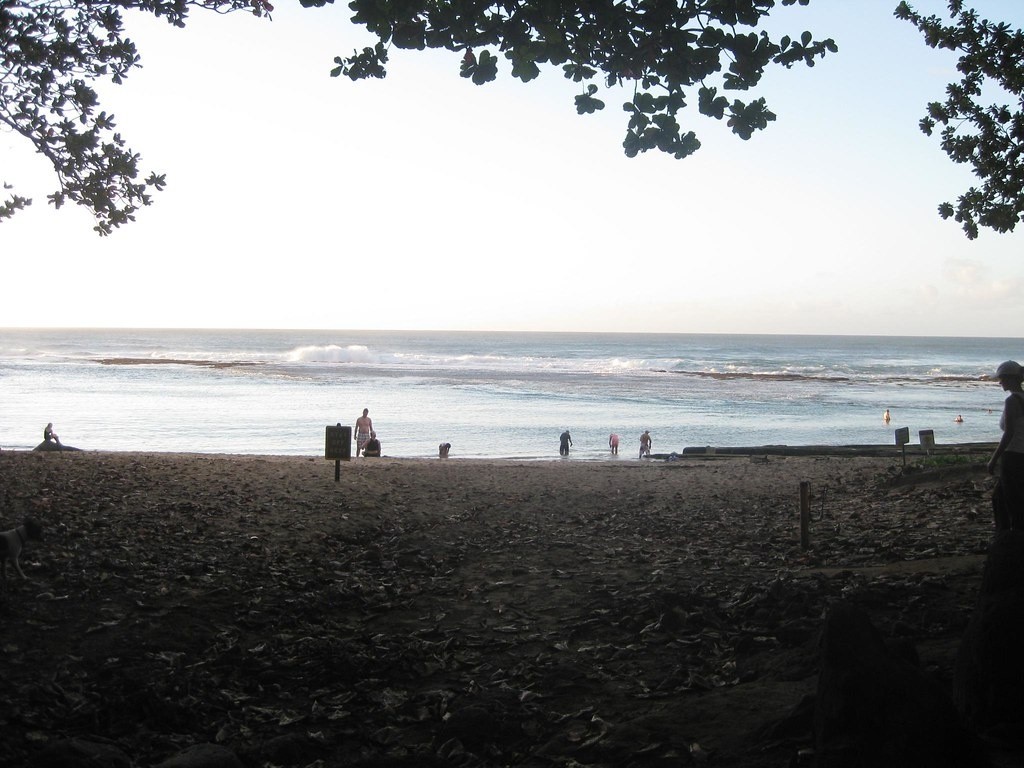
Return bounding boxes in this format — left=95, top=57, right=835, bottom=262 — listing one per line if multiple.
left=955, top=415, right=962, bottom=422
left=639, top=430, right=652, bottom=458
left=44, top=423, right=60, bottom=443
left=439, top=443, right=451, bottom=458
left=988, top=361, right=1024, bottom=533
left=609, top=433, right=618, bottom=454
left=354, top=408, right=381, bottom=457
left=560, top=430, right=572, bottom=455
left=884, top=409, right=891, bottom=424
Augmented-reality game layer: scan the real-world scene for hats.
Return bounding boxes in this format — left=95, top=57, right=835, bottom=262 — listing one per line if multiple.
left=991, top=360, right=1023, bottom=379
